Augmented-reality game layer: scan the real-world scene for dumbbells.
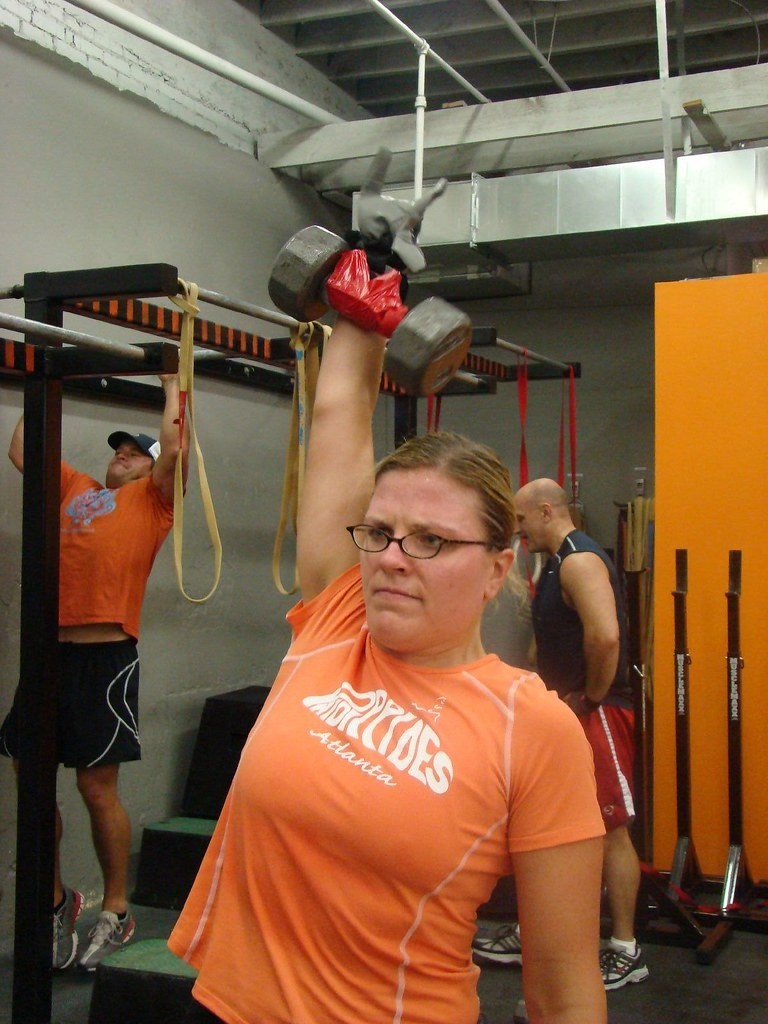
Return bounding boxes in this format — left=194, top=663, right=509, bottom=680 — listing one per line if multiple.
left=267, top=225, right=473, bottom=397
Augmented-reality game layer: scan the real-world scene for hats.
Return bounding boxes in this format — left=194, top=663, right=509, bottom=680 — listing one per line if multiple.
left=108, top=430, right=162, bottom=462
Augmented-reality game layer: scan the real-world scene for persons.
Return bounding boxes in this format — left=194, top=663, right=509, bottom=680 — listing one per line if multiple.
left=168, top=314, right=649, bottom=1024
left=7, top=354, right=191, bottom=975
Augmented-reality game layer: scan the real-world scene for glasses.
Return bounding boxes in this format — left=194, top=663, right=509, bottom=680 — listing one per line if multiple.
left=344, top=524, right=503, bottom=561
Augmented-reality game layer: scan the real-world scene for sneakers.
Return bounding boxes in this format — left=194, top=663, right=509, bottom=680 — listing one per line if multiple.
left=79, top=902, right=136, bottom=974
left=471, top=923, right=525, bottom=967
left=51, top=885, right=84, bottom=971
left=598, top=944, right=651, bottom=991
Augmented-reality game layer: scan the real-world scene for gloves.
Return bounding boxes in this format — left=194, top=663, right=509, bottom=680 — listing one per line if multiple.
left=342, top=228, right=411, bottom=308
